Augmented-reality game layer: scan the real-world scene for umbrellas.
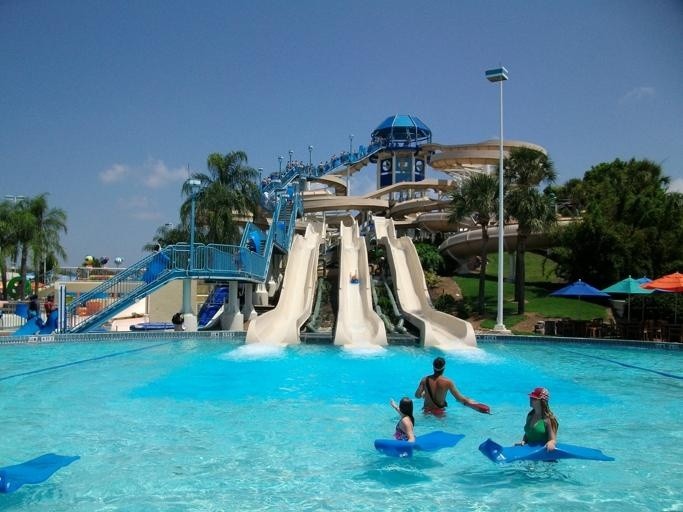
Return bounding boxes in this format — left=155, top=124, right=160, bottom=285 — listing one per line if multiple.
left=600, top=275, right=656, bottom=321
left=640, top=270, right=683, bottom=322
left=547, top=279, right=612, bottom=322
left=635, top=275, right=676, bottom=322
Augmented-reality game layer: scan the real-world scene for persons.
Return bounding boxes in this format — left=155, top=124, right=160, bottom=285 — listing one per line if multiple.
left=390, top=396, right=416, bottom=443
left=413, top=356, right=490, bottom=419
left=153, top=239, right=165, bottom=255
left=513, top=387, right=559, bottom=454
left=244, top=237, right=257, bottom=252
left=316, top=134, right=389, bottom=176
left=44, top=293, right=56, bottom=335
left=260, top=158, right=313, bottom=208
left=234, top=247, right=242, bottom=273
left=29, top=293, right=40, bottom=336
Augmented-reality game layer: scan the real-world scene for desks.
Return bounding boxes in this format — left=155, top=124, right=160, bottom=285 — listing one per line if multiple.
left=544, top=320, right=591, bottom=336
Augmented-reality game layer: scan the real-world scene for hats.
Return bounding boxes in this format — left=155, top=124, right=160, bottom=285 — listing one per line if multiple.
left=527, top=387, right=549, bottom=400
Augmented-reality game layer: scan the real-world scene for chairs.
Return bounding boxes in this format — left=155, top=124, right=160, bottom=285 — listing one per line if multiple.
left=585, top=316, right=603, bottom=337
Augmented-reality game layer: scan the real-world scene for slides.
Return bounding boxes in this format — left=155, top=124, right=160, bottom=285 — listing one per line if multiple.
left=130, top=285, right=242, bottom=330
left=333, top=221, right=388, bottom=348
left=12, top=304, right=58, bottom=336
left=371, top=217, right=477, bottom=347
left=244, top=222, right=328, bottom=345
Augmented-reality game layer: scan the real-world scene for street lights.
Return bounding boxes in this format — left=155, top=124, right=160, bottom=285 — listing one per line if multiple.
left=258, top=143, right=313, bottom=192
left=188, top=178, right=201, bottom=270
left=349, top=133, right=353, bottom=155
left=486, top=64, right=512, bottom=336
